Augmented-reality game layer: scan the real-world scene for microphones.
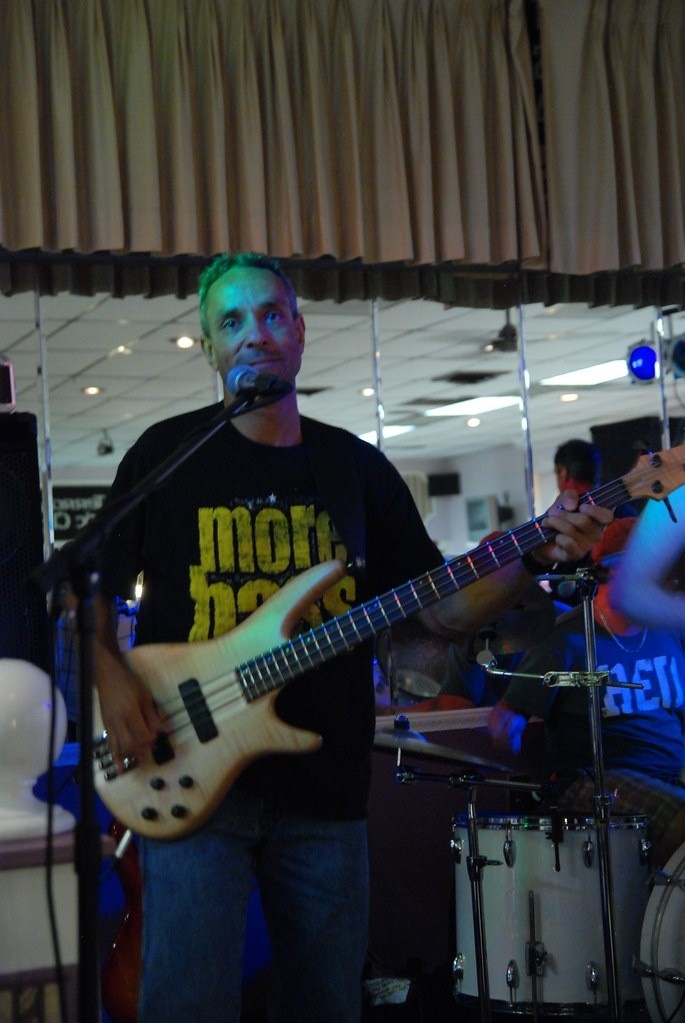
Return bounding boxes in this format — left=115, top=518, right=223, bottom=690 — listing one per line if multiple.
left=225, top=364, right=294, bottom=401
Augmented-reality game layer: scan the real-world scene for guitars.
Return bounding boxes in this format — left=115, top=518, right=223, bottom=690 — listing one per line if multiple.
left=86, top=442, right=685, bottom=845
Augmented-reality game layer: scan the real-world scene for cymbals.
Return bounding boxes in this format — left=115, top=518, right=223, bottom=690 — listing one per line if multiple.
left=372, top=711, right=517, bottom=776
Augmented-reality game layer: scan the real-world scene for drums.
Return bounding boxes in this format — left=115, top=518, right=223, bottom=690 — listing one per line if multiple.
left=637, top=839, right=685, bottom=1023
left=446, top=810, right=652, bottom=1019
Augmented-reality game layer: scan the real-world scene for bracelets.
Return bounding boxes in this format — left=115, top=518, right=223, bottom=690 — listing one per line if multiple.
left=521, top=552, right=569, bottom=576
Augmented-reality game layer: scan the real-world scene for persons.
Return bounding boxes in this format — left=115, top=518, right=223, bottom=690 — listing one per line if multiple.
left=376, top=440, right=685, bottom=869
left=65, top=254, right=615, bottom=1023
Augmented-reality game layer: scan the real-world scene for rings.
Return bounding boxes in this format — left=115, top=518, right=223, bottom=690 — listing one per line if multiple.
left=575, top=535, right=584, bottom=544
